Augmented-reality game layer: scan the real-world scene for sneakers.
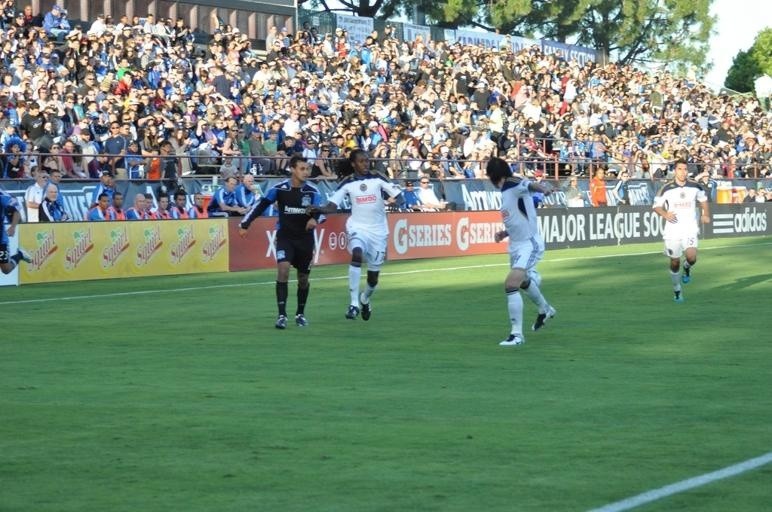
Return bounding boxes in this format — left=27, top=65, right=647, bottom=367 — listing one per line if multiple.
left=673, top=288, right=683, bottom=303
left=360, top=291, right=371, bottom=320
left=17, top=248, right=34, bottom=264
left=499, top=334, right=525, bottom=346
left=276, top=314, right=287, bottom=329
left=532, top=306, right=555, bottom=333
left=346, top=305, right=358, bottom=318
left=683, top=267, right=689, bottom=283
left=295, top=314, right=307, bottom=327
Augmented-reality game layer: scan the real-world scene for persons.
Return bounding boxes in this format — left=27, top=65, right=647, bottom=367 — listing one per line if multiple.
left=0, top=186, right=33, bottom=274
left=487, top=158, right=555, bottom=346
left=305, top=150, right=405, bottom=321
left=652, top=161, right=711, bottom=301
left=238, top=156, right=326, bottom=329
